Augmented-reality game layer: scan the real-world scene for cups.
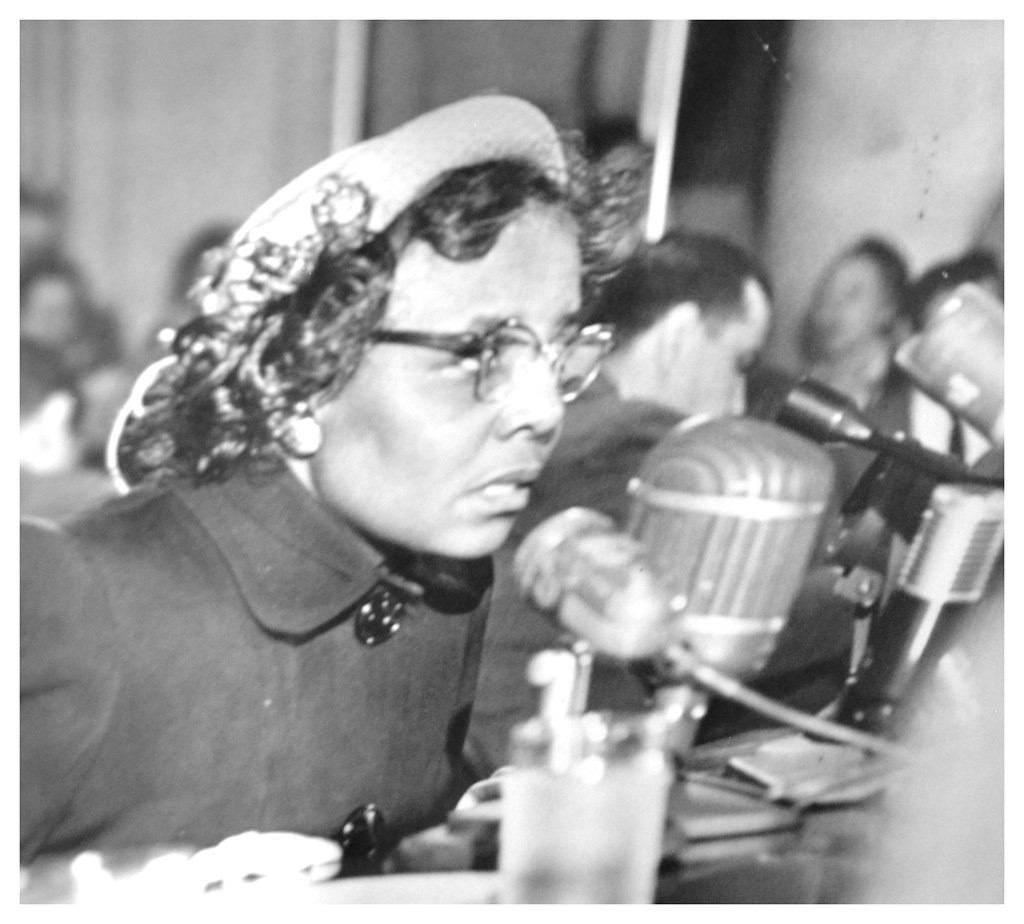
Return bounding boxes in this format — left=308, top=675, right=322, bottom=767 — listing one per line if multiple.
left=501, top=711, right=670, bottom=904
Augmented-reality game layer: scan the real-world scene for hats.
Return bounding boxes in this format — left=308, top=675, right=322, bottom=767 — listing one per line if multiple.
left=186, top=95, right=569, bottom=317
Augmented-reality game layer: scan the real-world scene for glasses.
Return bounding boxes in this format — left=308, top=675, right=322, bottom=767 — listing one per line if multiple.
left=368, top=314, right=624, bottom=404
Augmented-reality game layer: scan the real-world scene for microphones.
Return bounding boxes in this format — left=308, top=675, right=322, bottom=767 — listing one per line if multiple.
left=514, top=282, right=1005, bottom=768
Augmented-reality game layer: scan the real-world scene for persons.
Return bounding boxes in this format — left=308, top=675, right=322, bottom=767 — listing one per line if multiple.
left=20, top=93, right=649, bottom=905
left=20, top=120, right=1005, bottom=780
